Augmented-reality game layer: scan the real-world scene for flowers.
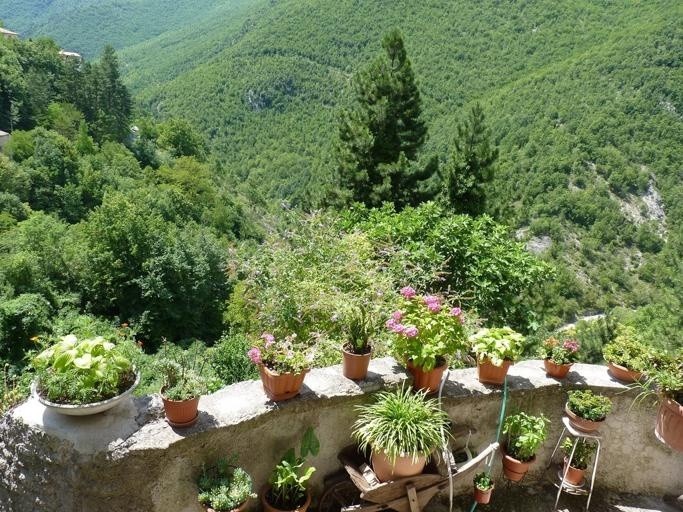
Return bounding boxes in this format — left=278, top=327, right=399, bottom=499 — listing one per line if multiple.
left=533, top=328, right=579, bottom=366
left=385, top=285, right=470, bottom=370
left=468, top=325, right=526, bottom=363
left=245, top=331, right=320, bottom=374
left=312, top=283, right=387, bottom=353
left=24, top=330, right=133, bottom=404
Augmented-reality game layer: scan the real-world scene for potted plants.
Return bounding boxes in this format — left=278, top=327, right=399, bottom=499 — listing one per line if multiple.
left=197, top=425, right=319, bottom=511
left=155, top=335, right=217, bottom=428
left=473, top=407, right=553, bottom=504
left=559, top=437, right=601, bottom=486
left=563, top=389, right=611, bottom=434
left=602, top=321, right=683, bottom=453
left=346, top=375, right=457, bottom=483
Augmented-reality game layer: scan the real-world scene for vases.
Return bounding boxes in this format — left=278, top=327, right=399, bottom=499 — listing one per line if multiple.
left=478, top=355, right=510, bottom=389
left=29, top=369, right=141, bottom=417
left=544, top=358, right=575, bottom=380
left=342, top=342, right=372, bottom=380
left=408, top=355, right=447, bottom=395
left=259, top=361, right=311, bottom=401
